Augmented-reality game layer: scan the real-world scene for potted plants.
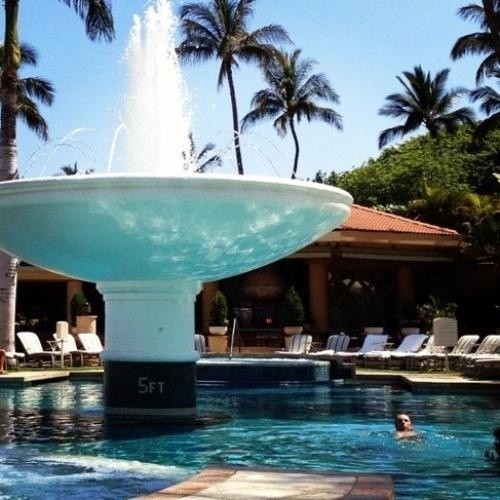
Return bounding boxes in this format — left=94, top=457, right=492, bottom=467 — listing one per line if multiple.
left=207, top=292, right=229, bottom=335
left=283, top=286, right=304, bottom=334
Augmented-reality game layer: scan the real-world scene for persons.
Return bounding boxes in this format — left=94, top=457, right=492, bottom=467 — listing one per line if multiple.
left=392, top=413, right=417, bottom=441
left=0, top=348, right=6, bottom=374
left=484, top=427, right=499, bottom=460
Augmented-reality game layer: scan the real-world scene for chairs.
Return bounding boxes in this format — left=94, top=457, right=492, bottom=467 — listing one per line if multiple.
left=15, top=319, right=104, bottom=367
left=273, top=327, right=499, bottom=378
